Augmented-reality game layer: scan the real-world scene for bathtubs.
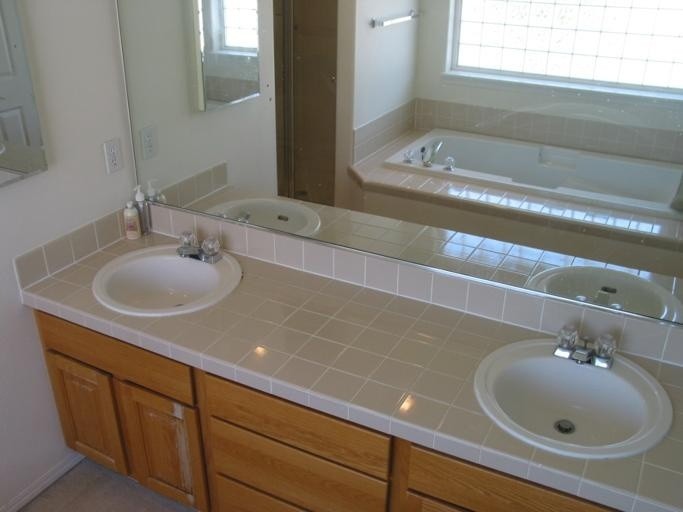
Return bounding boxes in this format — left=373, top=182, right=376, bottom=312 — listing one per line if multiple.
left=381, top=127, right=682, bottom=222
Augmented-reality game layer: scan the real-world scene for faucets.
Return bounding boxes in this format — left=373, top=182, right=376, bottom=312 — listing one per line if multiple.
left=235, top=208, right=251, bottom=224
left=572, top=335, right=595, bottom=366
left=175, top=231, right=201, bottom=259
left=593, top=291, right=610, bottom=306
left=421, top=138, right=444, bottom=166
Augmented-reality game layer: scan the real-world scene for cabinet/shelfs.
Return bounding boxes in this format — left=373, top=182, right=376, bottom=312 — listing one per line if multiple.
left=400, top=445, right=615, bottom=512
left=32, top=309, right=211, bottom=512
left=201, top=372, right=394, bottom=512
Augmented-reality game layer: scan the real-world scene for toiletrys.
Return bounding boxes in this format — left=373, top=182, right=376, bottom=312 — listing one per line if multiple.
left=133, top=184, right=151, bottom=235
left=123, top=201, right=142, bottom=239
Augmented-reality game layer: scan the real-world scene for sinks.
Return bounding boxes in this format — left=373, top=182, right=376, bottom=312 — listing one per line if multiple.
left=201, top=197, right=321, bottom=237
left=472, top=337, right=675, bottom=459
left=91, top=242, right=244, bottom=316
left=523, top=264, right=683, bottom=325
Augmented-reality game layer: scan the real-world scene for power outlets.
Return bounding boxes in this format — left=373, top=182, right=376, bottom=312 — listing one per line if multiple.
left=103, top=137, right=121, bottom=175
left=139, top=124, right=156, bottom=162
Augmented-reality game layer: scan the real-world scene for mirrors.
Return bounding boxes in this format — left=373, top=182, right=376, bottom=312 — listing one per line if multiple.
left=180, top=0, right=264, bottom=113
left=112, top=0, right=683, bottom=325
left=0, top=1, right=48, bottom=188
